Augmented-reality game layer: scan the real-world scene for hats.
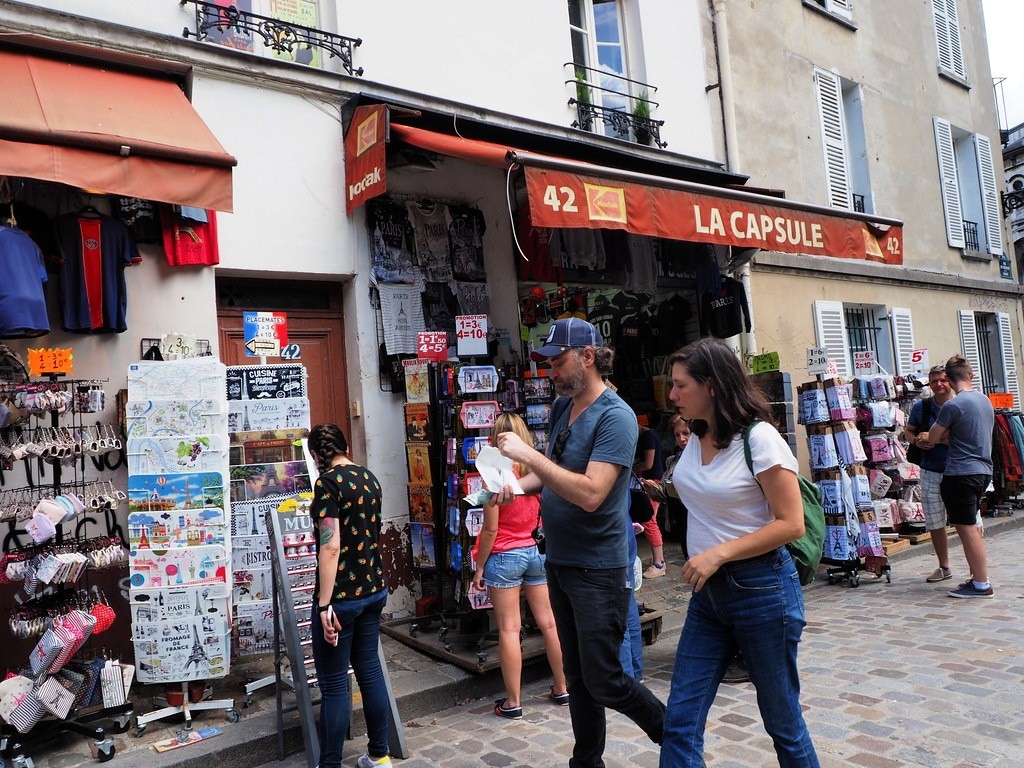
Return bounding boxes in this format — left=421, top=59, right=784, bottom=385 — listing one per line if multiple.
left=594, top=296, right=610, bottom=311
left=530, top=316, right=603, bottom=361
left=565, top=299, right=578, bottom=311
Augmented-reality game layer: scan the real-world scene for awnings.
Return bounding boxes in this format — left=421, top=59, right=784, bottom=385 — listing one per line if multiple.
left=388, top=123, right=903, bottom=266
left=0, top=46, right=237, bottom=214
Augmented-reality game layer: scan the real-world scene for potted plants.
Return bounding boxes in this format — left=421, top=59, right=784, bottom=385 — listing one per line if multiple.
left=574, top=70, right=593, bottom=132
left=630, top=94, right=652, bottom=145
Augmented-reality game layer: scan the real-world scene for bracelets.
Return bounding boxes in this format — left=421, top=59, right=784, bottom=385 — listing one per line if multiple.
left=914, top=439, right=916, bottom=445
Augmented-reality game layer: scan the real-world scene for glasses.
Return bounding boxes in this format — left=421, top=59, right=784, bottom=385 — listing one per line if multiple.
left=551, top=427, right=571, bottom=463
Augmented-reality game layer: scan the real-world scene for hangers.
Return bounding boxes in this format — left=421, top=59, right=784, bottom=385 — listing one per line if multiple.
left=78, top=196, right=101, bottom=217
left=380, top=193, right=474, bottom=214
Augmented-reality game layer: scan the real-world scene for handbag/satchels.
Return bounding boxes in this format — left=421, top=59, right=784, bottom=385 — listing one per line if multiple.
left=629, top=488, right=654, bottom=523
left=0, top=425, right=128, bottom=733
left=744, top=420, right=827, bottom=586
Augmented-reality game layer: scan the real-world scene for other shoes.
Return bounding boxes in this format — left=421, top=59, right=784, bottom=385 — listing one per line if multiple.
left=720, top=657, right=750, bottom=683
left=948, top=578, right=993, bottom=598
left=926, top=567, right=951, bottom=582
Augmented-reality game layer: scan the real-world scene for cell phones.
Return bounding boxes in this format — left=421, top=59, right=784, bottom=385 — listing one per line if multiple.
left=641, top=478, right=666, bottom=499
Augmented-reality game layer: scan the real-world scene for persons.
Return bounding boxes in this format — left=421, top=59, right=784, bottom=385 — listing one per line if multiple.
left=634, top=424, right=666, bottom=580
left=917, top=355, right=994, bottom=598
left=493, top=316, right=667, bottom=768
left=644, top=414, right=691, bottom=562
left=904, top=366, right=985, bottom=583
left=472, top=412, right=569, bottom=721
left=659, top=340, right=820, bottom=768
left=309, top=424, right=394, bottom=768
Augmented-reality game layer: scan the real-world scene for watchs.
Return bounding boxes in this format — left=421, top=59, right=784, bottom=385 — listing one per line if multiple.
left=316, top=604, right=331, bottom=614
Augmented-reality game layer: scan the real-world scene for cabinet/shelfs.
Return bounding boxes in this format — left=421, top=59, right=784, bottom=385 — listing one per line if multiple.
left=264, top=508, right=409, bottom=768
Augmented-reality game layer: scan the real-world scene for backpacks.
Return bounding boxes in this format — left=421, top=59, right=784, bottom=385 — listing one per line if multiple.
left=907, top=397, right=931, bottom=465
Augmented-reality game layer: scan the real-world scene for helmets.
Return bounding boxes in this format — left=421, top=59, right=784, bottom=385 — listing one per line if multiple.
left=528, top=287, right=544, bottom=301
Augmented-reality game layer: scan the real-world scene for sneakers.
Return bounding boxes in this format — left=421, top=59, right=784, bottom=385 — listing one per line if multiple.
left=549, top=685, right=569, bottom=706
left=643, top=560, right=667, bottom=579
left=494, top=697, right=523, bottom=719
left=358, top=752, right=393, bottom=768
